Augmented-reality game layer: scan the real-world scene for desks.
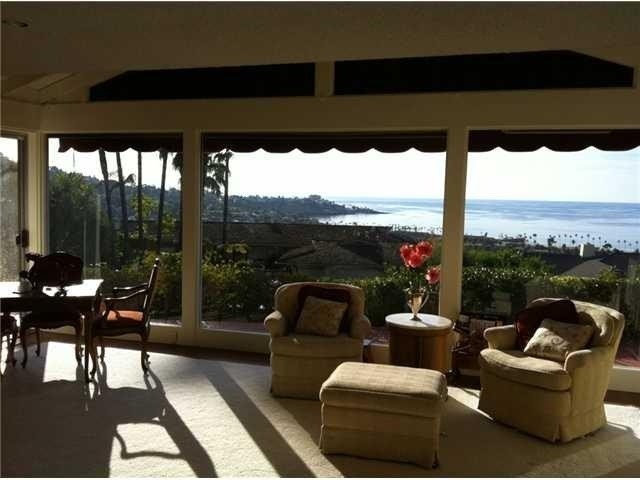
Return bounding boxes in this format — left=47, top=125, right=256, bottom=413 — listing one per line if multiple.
left=0, top=279, right=104, bottom=384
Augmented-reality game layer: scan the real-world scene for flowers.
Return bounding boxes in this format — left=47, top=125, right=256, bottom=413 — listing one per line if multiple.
left=400, top=240, right=440, bottom=292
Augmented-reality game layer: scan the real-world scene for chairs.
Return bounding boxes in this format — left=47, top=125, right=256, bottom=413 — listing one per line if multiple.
left=19, top=250, right=83, bottom=368
left=87, top=257, right=161, bottom=379
left=0, top=311, right=17, bottom=365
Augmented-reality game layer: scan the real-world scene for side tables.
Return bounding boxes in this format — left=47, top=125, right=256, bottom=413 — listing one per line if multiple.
left=386, top=313, right=455, bottom=374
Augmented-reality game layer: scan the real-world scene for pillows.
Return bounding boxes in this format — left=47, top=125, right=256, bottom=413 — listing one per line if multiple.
left=296, top=286, right=352, bottom=334
left=524, top=318, right=593, bottom=362
left=515, top=299, right=578, bottom=352
left=295, top=296, right=348, bottom=337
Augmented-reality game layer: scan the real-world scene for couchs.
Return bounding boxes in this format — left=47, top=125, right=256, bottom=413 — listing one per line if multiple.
left=477, top=297, right=626, bottom=443
left=264, top=282, right=372, bottom=400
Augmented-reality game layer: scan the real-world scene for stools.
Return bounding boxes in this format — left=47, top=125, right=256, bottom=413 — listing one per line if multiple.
left=319, top=361, right=448, bottom=468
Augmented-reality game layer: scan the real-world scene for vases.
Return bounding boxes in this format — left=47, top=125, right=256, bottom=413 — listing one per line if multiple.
left=404, top=292, right=429, bottom=321
